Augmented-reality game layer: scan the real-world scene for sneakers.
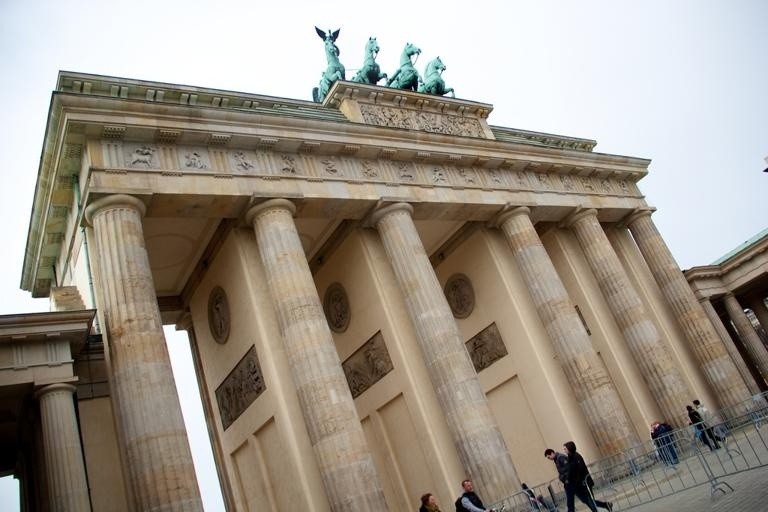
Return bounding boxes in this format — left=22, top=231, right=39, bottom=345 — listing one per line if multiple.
left=606, top=502, right=612, bottom=512
left=710, top=437, right=727, bottom=451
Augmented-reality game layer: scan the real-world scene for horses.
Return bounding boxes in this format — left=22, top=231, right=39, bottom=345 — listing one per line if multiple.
left=385, top=42, right=422, bottom=92
left=350, top=36, right=388, bottom=85
left=418, top=55, right=455, bottom=98
left=317, top=36, right=345, bottom=103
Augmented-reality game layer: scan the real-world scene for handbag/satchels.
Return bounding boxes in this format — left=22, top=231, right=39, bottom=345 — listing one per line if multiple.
left=693, top=425, right=702, bottom=438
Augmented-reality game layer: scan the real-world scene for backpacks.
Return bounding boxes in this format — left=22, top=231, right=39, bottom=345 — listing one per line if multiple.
left=455, top=497, right=470, bottom=512
left=661, top=422, right=673, bottom=437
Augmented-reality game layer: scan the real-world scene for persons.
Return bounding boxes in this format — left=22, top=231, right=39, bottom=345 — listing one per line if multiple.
left=544, top=448, right=577, bottom=512
left=687, top=405, right=727, bottom=451
left=650, top=418, right=680, bottom=464
left=689, top=423, right=705, bottom=448
left=693, top=400, right=726, bottom=438
left=419, top=493, right=441, bottom=512
left=522, top=482, right=539, bottom=511
left=562, top=441, right=613, bottom=512
left=455, top=479, right=497, bottom=512
